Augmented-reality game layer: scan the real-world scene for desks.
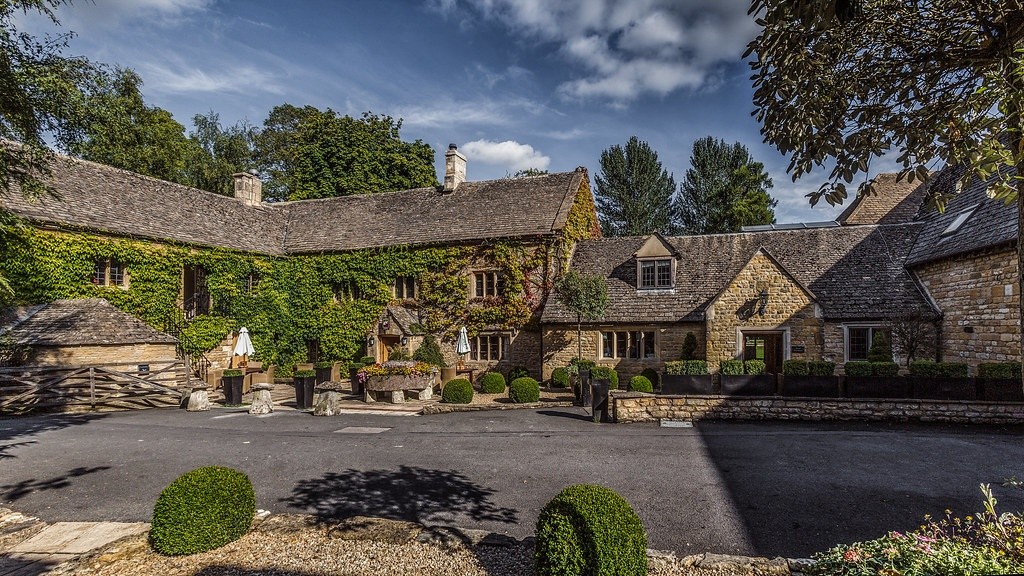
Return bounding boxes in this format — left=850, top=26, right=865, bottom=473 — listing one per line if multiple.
left=246, top=368, right=260, bottom=373
left=457, top=368, right=480, bottom=385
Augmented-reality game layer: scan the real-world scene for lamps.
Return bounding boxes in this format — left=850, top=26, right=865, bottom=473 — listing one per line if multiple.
left=402, top=333, right=407, bottom=346
left=369, top=334, right=375, bottom=346
left=758, top=287, right=768, bottom=315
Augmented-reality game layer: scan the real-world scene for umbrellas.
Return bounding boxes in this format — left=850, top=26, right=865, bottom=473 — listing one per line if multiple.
left=233, top=326, right=255, bottom=368
left=455, top=327, right=471, bottom=367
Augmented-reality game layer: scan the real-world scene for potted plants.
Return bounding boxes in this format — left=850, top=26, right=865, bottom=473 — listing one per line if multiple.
left=348, top=356, right=377, bottom=396
left=838, top=361, right=1024, bottom=402
left=661, top=360, right=714, bottom=395
left=222, top=369, right=244, bottom=406
left=777, top=360, right=840, bottom=397
left=578, top=359, right=621, bottom=424
left=314, top=362, right=332, bottom=387
left=720, top=361, right=776, bottom=396
left=294, top=371, right=316, bottom=409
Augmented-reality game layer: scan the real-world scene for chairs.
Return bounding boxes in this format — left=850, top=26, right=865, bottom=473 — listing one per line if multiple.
left=233, top=361, right=276, bottom=395
left=441, top=368, right=456, bottom=389
